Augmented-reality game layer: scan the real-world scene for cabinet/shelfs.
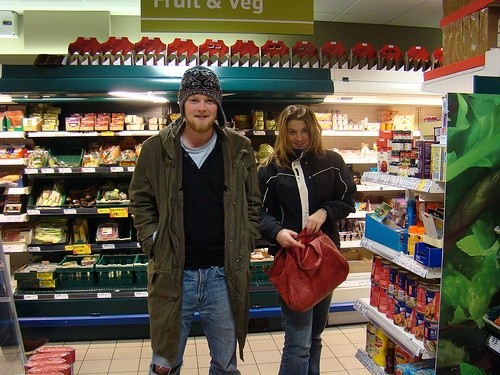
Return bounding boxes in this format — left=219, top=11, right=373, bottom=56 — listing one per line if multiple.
left=0, top=92, right=324, bottom=341
left=350, top=170, right=446, bottom=375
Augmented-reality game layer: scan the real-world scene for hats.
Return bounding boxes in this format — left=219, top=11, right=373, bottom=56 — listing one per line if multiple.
left=179, top=65, right=227, bottom=129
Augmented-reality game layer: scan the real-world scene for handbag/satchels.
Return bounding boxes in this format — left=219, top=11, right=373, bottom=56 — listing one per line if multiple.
left=267, top=226, right=349, bottom=311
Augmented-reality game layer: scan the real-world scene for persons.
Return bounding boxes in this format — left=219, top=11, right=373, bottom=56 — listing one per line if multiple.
left=258, top=104, right=358, bottom=375
left=128, top=67, right=262, bottom=375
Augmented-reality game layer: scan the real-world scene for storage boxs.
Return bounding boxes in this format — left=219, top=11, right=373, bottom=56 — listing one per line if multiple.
left=0, top=105, right=126, bottom=133
left=16, top=280, right=58, bottom=291
left=27, top=176, right=67, bottom=208
left=95, top=254, right=136, bottom=287
left=364, top=211, right=408, bottom=252
left=95, top=181, right=130, bottom=207
left=0, top=217, right=132, bottom=243
left=413, top=239, right=442, bottom=268
left=414, top=93, right=457, bottom=182
left=134, top=253, right=150, bottom=287
left=14, top=263, right=57, bottom=280
left=249, top=257, right=275, bottom=282
left=43, top=145, right=84, bottom=167
left=55, top=254, right=101, bottom=290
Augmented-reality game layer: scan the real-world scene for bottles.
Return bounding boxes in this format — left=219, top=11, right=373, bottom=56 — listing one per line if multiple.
left=365, top=321, right=395, bottom=375
